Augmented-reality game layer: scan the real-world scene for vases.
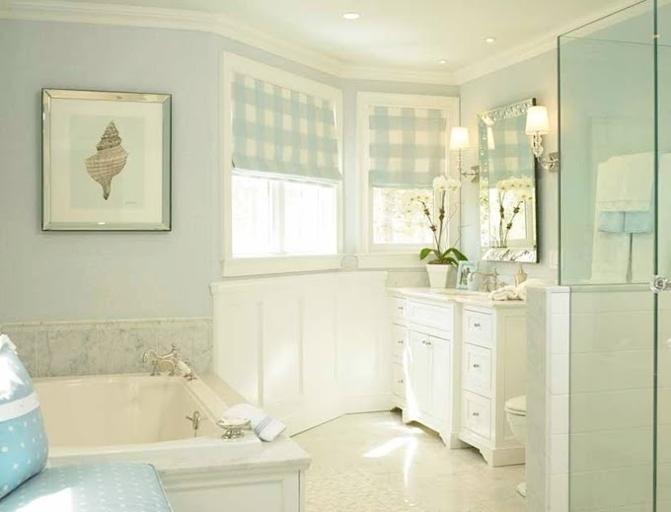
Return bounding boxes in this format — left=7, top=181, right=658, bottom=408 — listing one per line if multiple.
left=426, top=262, right=458, bottom=288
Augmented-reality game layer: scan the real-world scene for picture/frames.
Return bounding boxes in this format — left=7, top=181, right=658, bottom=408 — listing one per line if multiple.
left=40, top=88, right=172, bottom=235
left=455, top=259, right=479, bottom=290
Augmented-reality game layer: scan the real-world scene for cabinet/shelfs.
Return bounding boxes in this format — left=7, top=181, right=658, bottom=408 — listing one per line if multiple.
left=389, top=295, right=527, bottom=469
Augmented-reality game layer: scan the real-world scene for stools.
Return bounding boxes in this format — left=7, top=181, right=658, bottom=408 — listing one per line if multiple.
left=0, top=458, right=170, bottom=510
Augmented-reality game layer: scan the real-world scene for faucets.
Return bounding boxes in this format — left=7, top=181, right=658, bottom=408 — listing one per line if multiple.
left=469, top=267, right=499, bottom=290
left=143, top=342, right=180, bottom=375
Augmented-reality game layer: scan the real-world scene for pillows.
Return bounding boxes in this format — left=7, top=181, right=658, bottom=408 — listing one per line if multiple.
left=0, top=331, right=49, bottom=502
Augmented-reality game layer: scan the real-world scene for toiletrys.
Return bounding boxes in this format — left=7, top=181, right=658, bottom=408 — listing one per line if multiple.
left=514, top=260, right=528, bottom=285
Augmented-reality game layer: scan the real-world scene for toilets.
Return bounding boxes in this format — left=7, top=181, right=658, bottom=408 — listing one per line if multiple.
left=505, top=395, right=527, bottom=497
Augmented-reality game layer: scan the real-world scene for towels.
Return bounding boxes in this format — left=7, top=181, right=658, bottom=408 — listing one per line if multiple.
left=225, top=402, right=284, bottom=442
left=581, top=152, right=668, bottom=282
left=490, top=279, right=543, bottom=302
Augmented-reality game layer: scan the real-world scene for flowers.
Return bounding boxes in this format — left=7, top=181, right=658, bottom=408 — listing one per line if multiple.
left=492, top=175, right=533, bottom=245
left=406, top=175, right=468, bottom=265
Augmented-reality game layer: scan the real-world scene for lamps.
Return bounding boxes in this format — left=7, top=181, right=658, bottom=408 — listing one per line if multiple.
left=523, top=105, right=560, bottom=173
left=448, top=125, right=477, bottom=183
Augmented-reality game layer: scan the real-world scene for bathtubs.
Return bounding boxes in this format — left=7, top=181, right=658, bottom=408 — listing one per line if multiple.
left=29, top=366, right=262, bottom=454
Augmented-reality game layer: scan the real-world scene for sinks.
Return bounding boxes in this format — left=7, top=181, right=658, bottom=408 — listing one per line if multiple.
left=437, top=289, right=487, bottom=295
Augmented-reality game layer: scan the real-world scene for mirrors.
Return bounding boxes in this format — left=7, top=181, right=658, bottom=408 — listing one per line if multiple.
left=474, top=99, right=540, bottom=264
